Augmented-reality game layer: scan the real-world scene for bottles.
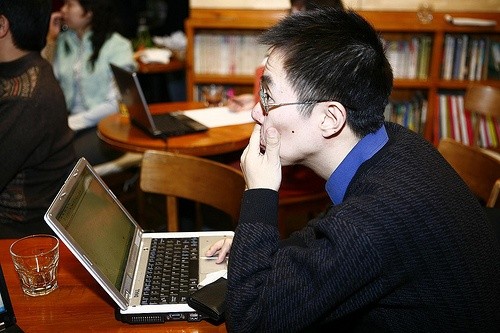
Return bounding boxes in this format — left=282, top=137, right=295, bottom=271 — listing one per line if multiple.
left=136, top=19, right=154, bottom=49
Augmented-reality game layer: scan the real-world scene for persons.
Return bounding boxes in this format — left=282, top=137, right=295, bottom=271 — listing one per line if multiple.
left=0, top=0, right=74, bottom=235
left=207, top=8, right=500, bottom=333
left=40, top=0, right=136, bottom=168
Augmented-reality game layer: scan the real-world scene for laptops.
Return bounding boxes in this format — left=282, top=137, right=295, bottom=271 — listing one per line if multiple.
left=43, top=157, right=235, bottom=324
left=110, top=63, right=209, bottom=138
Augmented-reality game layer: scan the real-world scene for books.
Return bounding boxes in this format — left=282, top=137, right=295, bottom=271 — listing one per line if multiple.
left=195, top=28, right=500, bottom=149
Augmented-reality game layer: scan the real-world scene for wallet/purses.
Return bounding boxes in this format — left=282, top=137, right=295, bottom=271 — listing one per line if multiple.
left=187, top=277, right=229, bottom=326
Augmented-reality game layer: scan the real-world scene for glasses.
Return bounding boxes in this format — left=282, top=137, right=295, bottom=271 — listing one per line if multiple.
left=259, top=87, right=357, bottom=115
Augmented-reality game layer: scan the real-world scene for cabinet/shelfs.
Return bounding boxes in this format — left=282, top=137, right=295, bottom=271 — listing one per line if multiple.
left=183, top=11, right=499, bottom=149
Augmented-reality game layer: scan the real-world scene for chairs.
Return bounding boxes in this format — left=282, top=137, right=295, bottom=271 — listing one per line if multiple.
left=140, top=149, right=247, bottom=233
left=438, top=83, right=499, bottom=207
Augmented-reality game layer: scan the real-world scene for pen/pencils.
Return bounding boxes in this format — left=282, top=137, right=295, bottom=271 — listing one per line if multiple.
left=225, top=95, right=243, bottom=107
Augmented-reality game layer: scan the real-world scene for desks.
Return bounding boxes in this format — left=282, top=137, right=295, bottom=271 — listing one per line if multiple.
left=135, top=44, right=182, bottom=73
left=96, top=101, right=258, bottom=230
left=1, top=236, right=231, bottom=332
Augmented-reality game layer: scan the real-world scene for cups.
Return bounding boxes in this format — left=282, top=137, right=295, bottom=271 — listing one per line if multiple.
left=116, top=97, right=130, bottom=118
left=202, top=84, right=225, bottom=109
left=9, top=234, right=60, bottom=297
